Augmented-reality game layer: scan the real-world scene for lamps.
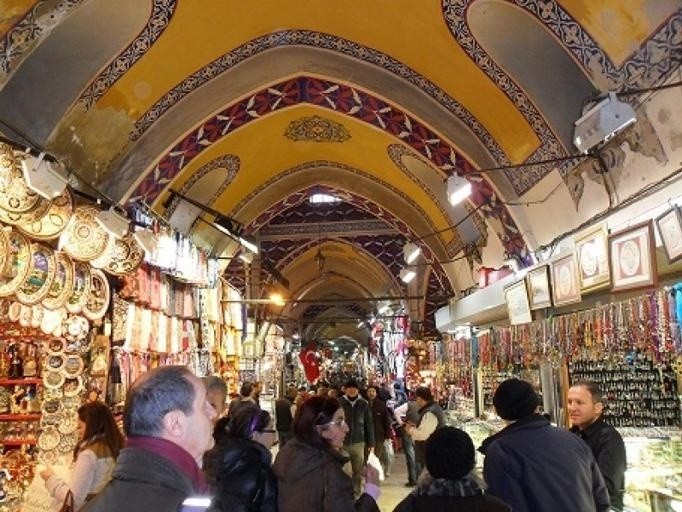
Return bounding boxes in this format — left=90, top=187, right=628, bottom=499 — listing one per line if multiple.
left=443, top=150, right=594, bottom=208
left=163, top=187, right=233, bottom=236
left=398, top=250, right=475, bottom=284
left=572, top=81, right=682, bottom=154
left=403, top=196, right=489, bottom=265
left=313, top=218, right=325, bottom=272
left=172, top=188, right=260, bottom=256
left=191, top=216, right=255, bottom=263
left=1, top=129, right=70, bottom=201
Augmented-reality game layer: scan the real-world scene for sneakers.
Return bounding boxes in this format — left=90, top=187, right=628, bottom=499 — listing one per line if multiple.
left=404, top=479, right=416, bottom=488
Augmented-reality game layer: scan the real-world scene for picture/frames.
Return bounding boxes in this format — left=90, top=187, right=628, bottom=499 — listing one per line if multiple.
left=551, top=251, right=582, bottom=309
left=656, top=203, right=682, bottom=264
left=607, top=217, right=659, bottom=295
left=574, top=223, right=613, bottom=294
left=526, top=262, right=554, bottom=311
left=503, top=275, right=536, bottom=326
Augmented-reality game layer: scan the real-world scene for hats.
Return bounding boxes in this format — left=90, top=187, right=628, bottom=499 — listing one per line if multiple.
left=416, top=387, right=434, bottom=401
left=491, top=378, right=540, bottom=422
left=423, top=424, right=477, bottom=481
left=345, top=379, right=358, bottom=389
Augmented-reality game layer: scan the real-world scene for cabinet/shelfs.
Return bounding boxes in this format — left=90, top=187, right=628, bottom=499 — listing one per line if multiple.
left=0, top=377, right=46, bottom=446
